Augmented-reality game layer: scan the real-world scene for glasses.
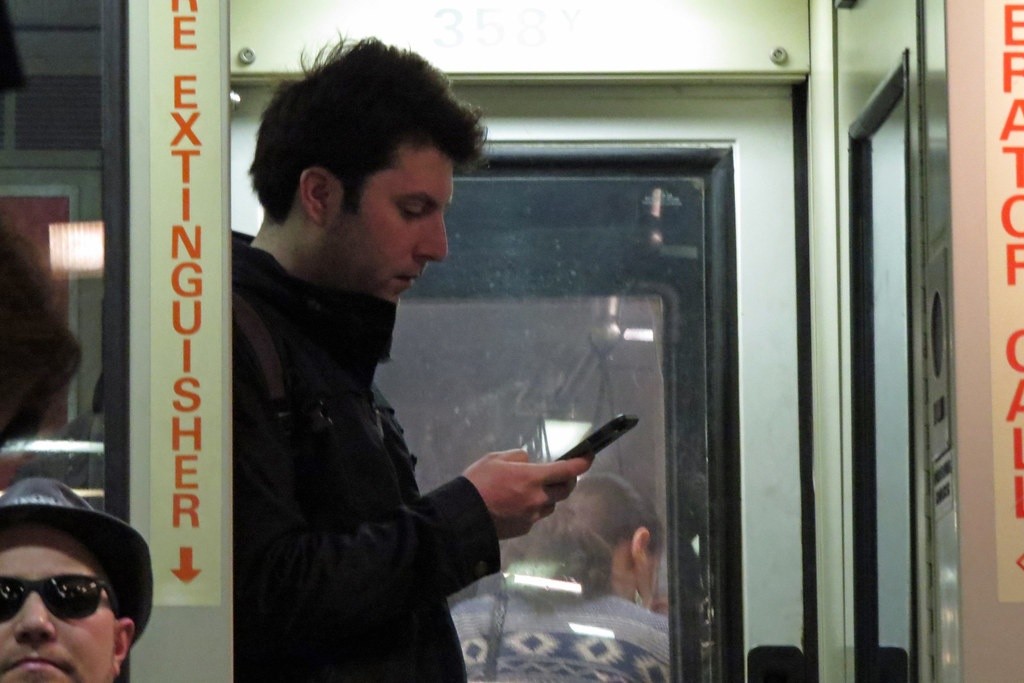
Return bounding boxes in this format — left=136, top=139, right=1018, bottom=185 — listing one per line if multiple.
left=0, top=574, right=120, bottom=623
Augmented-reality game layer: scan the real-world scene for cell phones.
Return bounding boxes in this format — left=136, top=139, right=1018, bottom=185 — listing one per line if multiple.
left=557, top=414, right=639, bottom=462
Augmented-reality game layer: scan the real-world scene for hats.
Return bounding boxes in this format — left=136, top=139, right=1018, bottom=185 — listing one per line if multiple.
left=0, top=477, right=153, bottom=648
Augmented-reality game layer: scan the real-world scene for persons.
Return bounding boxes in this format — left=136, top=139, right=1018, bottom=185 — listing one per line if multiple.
left=0, top=226, right=83, bottom=464
left=0, top=476, right=153, bottom=683
left=444, top=463, right=675, bottom=683
left=217, top=33, right=595, bottom=683
